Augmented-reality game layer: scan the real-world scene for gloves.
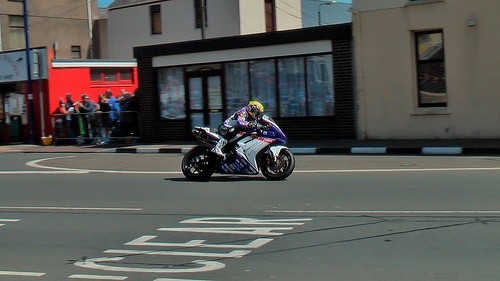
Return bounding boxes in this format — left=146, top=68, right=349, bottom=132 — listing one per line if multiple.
left=257, top=123, right=269, bottom=131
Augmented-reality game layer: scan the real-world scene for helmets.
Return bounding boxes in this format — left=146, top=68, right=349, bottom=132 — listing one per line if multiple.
left=247, top=101, right=263, bottom=119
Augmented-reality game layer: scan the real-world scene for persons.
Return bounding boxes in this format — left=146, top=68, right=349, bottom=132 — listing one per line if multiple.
left=211, top=101, right=264, bottom=157
left=54, top=87, right=139, bottom=147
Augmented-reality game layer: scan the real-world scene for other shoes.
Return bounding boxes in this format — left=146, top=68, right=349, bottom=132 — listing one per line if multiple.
left=90, top=140, right=118, bottom=145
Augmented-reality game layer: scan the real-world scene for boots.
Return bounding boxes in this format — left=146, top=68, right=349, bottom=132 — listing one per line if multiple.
left=211, top=138, right=228, bottom=157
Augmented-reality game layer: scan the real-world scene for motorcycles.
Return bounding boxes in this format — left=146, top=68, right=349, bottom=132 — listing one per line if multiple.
left=181, top=114, right=295, bottom=181
